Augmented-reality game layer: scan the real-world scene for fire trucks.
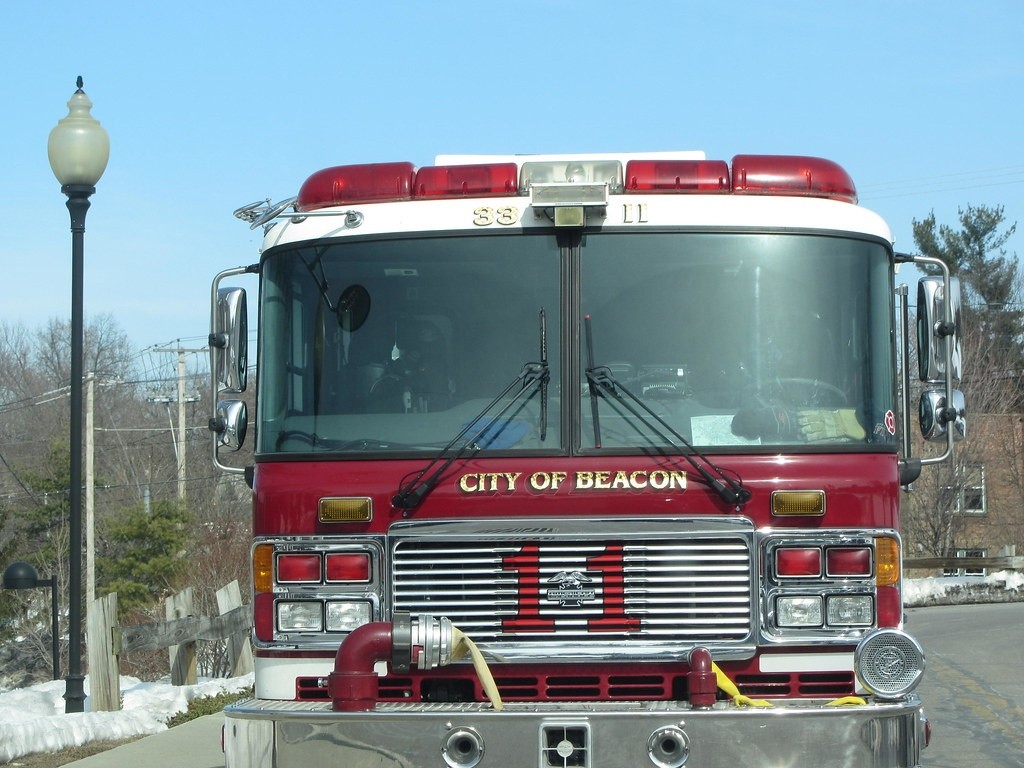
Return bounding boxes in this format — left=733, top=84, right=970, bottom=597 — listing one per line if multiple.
left=206, top=151, right=967, bottom=768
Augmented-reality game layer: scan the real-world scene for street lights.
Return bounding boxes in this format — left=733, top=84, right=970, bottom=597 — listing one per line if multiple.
left=2, top=563, right=59, bottom=682
left=45, top=75, right=112, bottom=712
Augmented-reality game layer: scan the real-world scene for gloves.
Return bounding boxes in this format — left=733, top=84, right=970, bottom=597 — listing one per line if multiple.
left=732, top=405, right=798, bottom=442
left=795, top=408, right=866, bottom=444
